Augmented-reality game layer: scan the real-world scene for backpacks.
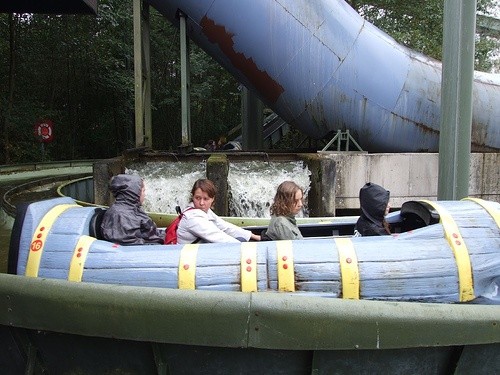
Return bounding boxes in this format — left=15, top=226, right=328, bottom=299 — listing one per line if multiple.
left=164, top=205, right=201, bottom=245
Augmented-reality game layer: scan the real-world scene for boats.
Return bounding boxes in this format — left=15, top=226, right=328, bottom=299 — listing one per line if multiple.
left=7, top=196, right=500, bottom=305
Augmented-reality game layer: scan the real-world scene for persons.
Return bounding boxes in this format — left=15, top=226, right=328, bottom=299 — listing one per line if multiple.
left=171, top=179, right=261, bottom=245
left=206, top=137, right=227, bottom=150
left=100, top=174, right=165, bottom=246
left=354, top=182, right=391, bottom=237
left=262, top=180, right=305, bottom=241
